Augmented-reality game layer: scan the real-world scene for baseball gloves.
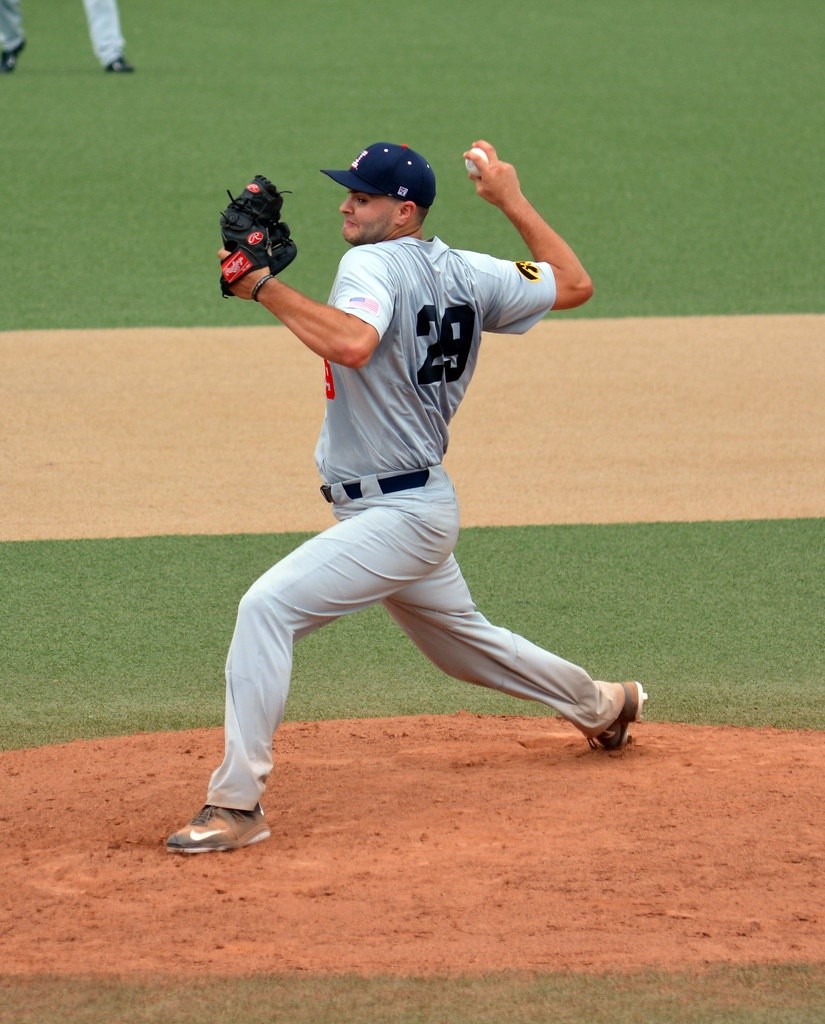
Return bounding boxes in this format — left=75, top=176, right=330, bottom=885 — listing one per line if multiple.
left=216, top=174, right=303, bottom=299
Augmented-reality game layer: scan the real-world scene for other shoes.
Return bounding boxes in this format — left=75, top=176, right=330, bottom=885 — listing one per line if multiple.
left=0, top=41, right=26, bottom=72
left=106, top=58, right=133, bottom=72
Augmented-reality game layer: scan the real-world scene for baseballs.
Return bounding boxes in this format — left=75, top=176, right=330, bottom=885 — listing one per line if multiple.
left=465, top=148, right=491, bottom=176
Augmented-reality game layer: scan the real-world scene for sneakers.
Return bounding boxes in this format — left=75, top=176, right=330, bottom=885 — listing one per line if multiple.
left=587, top=682, right=647, bottom=751
left=166, top=802, right=271, bottom=854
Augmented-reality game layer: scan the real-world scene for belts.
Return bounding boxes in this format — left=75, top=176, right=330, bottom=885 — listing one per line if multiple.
left=320, top=469, right=429, bottom=503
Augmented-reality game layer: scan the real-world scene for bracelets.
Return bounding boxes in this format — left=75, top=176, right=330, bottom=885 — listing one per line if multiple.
left=251, top=274, right=274, bottom=302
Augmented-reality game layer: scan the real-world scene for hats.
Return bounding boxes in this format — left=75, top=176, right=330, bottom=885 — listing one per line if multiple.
left=320, top=142, right=437, bottom=208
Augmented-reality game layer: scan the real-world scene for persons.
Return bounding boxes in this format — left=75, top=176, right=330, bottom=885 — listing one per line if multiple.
left=163, top=140, right=645, bottom=857
left=0, top=0, right=134, bottom=73
